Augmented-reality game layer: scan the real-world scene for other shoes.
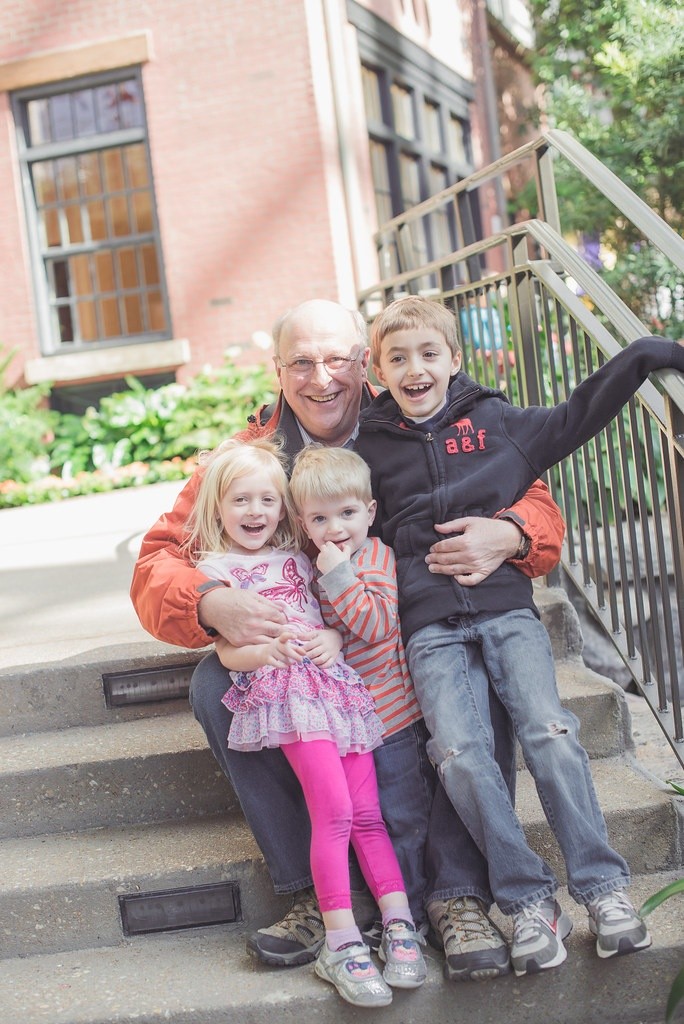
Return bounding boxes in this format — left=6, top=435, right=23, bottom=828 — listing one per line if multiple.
left=361, top=919, right=430, bottom=952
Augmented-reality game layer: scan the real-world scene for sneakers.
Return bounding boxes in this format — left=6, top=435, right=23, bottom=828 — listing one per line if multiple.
left=314, top=943, right=393, bottom=1008
left=427, top=897, right=512, bottom=985
left=247, top=887, right=372, bottom=967
left=585, top=888, right=653, bottom=958
left=512, top=896, right=574, bottom=977
left=378, top=925, right=427, bottom=989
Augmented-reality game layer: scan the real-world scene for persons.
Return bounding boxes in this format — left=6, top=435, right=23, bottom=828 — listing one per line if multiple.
left=130, top=300, right=566, bottom=981
left=176, top=426, right=428, bottom=1007
left=289, top=442, right=430, bottom=953
left=353, top=297, right=684, bottom=976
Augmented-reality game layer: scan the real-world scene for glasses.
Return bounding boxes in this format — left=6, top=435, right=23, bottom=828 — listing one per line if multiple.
left=278, top=350, right=361, bottom=377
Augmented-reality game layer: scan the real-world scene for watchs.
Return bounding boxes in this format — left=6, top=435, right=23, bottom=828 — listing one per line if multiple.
left=512, top=532, right=531, bottom=560
